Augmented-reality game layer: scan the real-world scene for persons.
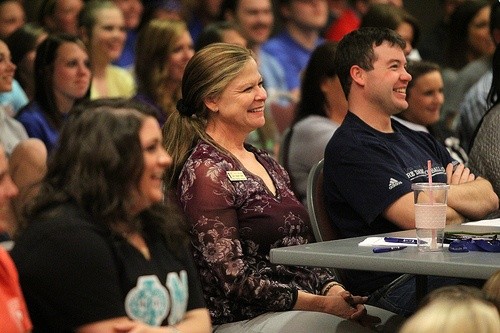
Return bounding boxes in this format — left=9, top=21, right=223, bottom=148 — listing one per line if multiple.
left=219, top=0, right=291, bottom=154
left=416, top=0, right=500, bottom=220
left=195, top=22, right=250, bottom=57
left=395, top=61, right=470, bottom=167
left=10, top=138, right=47, bottom=207
left=1, top=0, right=221, bottom=102
left=279, top=43, right=348, bottom=210
left=360, top=5, right=421, bottom=57
left=14, top=32, right=93, bottom=152
left=261, top=0, right=331, bottom=91
left=131, top=19, right=196, bottom=126
left=0, top=142, right=33, bottom=333
left=0, top=40, right=29, bottom=156
left=323, top=27, right=500, bottom=239
left=399, top=271, right=500, bottom=333
left=77, top=0, right=136, bottom=101
left=161, top=43, right=405, bottom=333
left=324, top=0, right=404, bottom=43
left=12, top=97, right=212, bottom=333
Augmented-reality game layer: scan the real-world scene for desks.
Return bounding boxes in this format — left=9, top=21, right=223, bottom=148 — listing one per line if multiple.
left=270, top=218, right=500, bottom=309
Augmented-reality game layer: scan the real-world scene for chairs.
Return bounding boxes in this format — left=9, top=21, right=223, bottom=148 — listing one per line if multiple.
left=308, top=157, right=340, bottom=277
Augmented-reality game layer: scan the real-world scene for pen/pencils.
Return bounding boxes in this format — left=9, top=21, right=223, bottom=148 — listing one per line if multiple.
left=344, top=295, right=358, bottom=309
left=384, top=236, right=427, bottom=244
left=373, top=245, right=407, bottom=253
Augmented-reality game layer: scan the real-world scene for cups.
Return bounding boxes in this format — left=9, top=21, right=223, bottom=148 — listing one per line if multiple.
left=411, top=183, right=451, bottom=252
